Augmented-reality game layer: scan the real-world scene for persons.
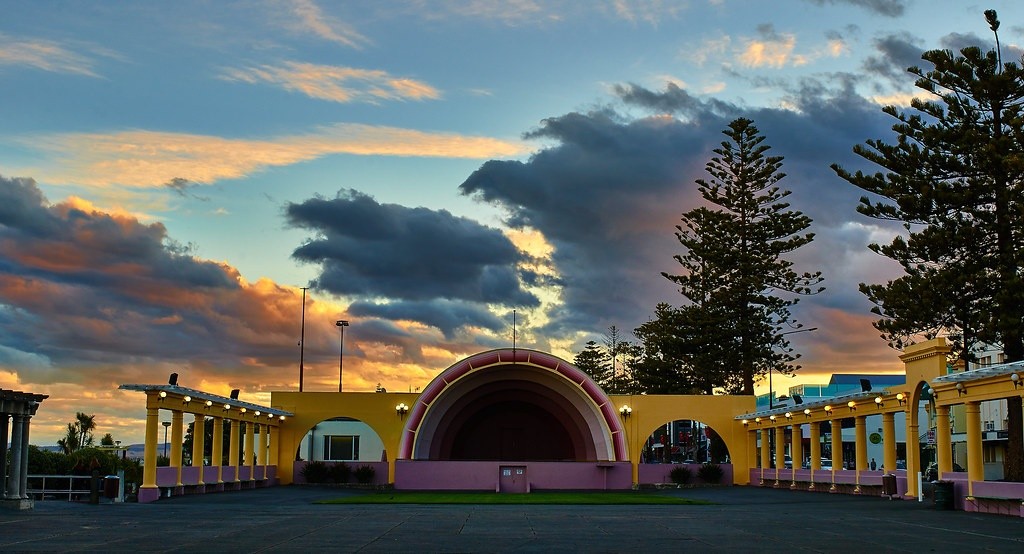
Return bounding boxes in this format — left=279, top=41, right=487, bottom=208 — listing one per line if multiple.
left=870, top=458, right=877, bottom=471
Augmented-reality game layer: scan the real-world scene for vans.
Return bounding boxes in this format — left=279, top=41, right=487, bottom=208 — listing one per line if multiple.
left=806, top=457, right=829, bottom=469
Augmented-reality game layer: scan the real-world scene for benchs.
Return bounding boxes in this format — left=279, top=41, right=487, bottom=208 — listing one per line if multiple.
left=965, top=495, right=1023, bottom=517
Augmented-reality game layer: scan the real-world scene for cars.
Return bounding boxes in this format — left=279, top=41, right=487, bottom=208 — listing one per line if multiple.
left=773, top=454, right=792, bottom=467
left=821, top=460, right=847, bottom=470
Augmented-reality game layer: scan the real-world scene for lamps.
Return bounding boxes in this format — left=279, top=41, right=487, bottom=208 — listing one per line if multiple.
left=955, top=382, right=967, bottom=396
left=222, top=404, right=231, bottom=414
left=253, top=411, right=261, bottom=420
left=896, top=393, right=906, bottom=406
left=238, top=408, right=247, bottom=418
left=804, top=409, right=812, bottom=419
left=183, top=396, right=192, bottom=408
left=157, top=391, right=167, bottom=404
left=755, top=417, right=762, bottom=426
left=847, top=401, right=857, bottom=412
left=204, top=401, right=213, bottom=411
left=618, top=405, right=632, bottom=423
left=742, top=419, right=748, bottom=427
left=875, top=397, right=884, bottom=410
left=927, top=388, right=938, bottom=399
left=395, top=402, right=409, bottom=421
left=278, top=415, right=285, bottom=424
left=769, top=415, right=776, bottom=424
left=267, top=413, right=274, bottom=422
left=1010, top=373, right=1023, bottom=390
left=785, top=413, right=793, bottom=422
left=824, top=406, right=832, bottom=416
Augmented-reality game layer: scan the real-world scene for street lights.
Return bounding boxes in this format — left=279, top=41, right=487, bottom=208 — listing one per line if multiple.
left=336, top=320, right=350, bottom=393
left=299, top=287, right=310, bottom=392
left=769, top=327, right=818, bottom=468
left=161, top=421, right=171, bottom=458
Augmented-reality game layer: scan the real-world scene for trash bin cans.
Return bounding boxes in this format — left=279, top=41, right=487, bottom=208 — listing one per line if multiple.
left=881, top=472, right=897, bottom=496
left=103, top=475, right=120, bottom=498
